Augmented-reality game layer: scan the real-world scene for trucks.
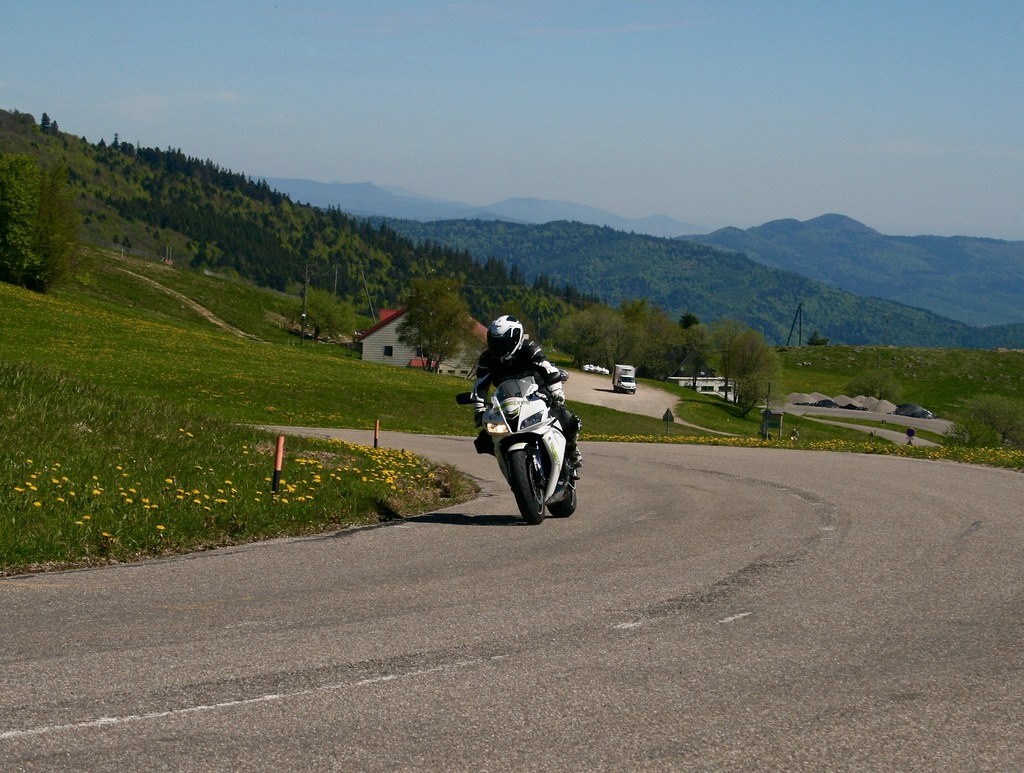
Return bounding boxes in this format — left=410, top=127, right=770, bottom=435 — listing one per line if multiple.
left=612, top=364, right=636, bottom=394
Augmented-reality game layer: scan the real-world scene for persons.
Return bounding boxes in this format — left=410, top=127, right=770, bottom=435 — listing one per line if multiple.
left=474, top=315, right=584, bottom=480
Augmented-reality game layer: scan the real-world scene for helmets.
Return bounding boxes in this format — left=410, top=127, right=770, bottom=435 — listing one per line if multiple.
left=488, top=315, right=524, bottom=363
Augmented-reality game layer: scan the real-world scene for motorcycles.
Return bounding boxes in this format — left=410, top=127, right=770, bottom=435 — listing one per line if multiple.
left=455, top=370, right=582, bottom=525
